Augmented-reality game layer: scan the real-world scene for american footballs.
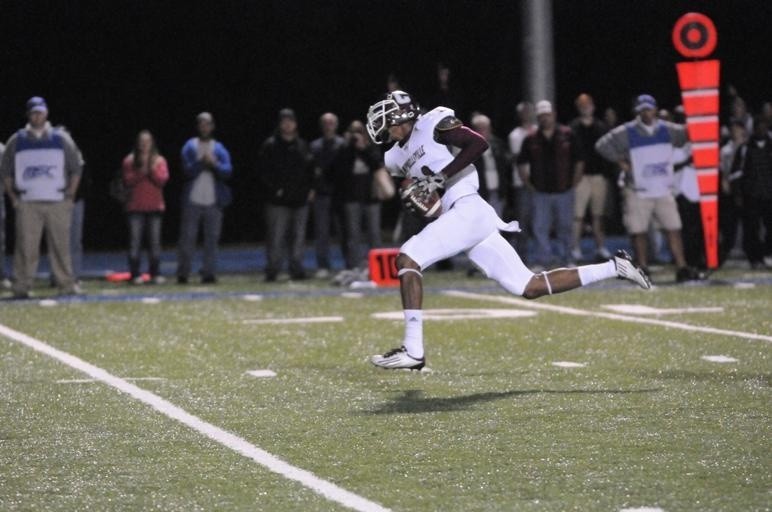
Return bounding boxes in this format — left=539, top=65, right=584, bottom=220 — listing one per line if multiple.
left=401, top=179, right=443, bottom=218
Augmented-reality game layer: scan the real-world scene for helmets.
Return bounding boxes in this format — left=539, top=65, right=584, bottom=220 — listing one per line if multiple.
left=366, top=90, right=421, bottom=145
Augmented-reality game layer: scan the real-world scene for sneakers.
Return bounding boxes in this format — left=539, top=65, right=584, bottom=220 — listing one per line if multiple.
left=613, top=249, right=651, bottom=289
left=371, top=345, right=425, bottom=370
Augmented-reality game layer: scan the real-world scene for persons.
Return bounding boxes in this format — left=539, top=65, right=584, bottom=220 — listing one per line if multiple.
left=55, top=125, right=91, bottom=285
left=124, top=128, right=169, bottom=286
left=0, top=140, right=11, bottom=286
left=173, top=111, right=232, bottom=286
left=259, top=93, right=772, bottom=285
left=1, top=96, right=87, bottom=300
left=366, top=91, right=652, bottom=371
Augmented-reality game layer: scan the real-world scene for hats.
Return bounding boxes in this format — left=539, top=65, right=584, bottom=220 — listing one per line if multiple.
left=635, top=95, right=656, bottom=112
left=28, top=97, right=47, bottom=112
left=535, top=101, right=552, bottom=116
